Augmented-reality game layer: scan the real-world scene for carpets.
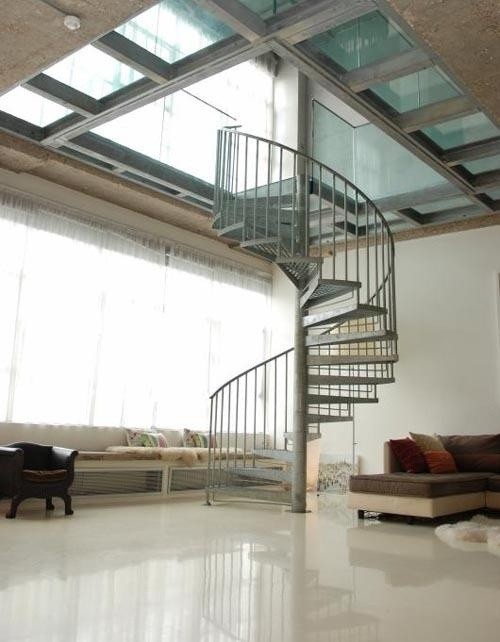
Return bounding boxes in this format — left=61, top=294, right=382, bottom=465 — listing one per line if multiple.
left=433, top=514, right=500, bottom=558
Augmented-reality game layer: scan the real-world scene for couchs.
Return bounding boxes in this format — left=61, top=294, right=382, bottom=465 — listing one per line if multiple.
left=344, top=434, right=500, bottom=525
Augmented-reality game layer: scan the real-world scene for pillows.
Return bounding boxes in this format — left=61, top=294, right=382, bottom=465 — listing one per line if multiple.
left=389, top=432, right=459, bottom=474
left=124, top=426, right=219, bottom=448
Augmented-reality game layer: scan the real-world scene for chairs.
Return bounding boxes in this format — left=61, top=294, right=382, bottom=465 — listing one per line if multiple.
left=0, top=441, right=81, bottom=518
